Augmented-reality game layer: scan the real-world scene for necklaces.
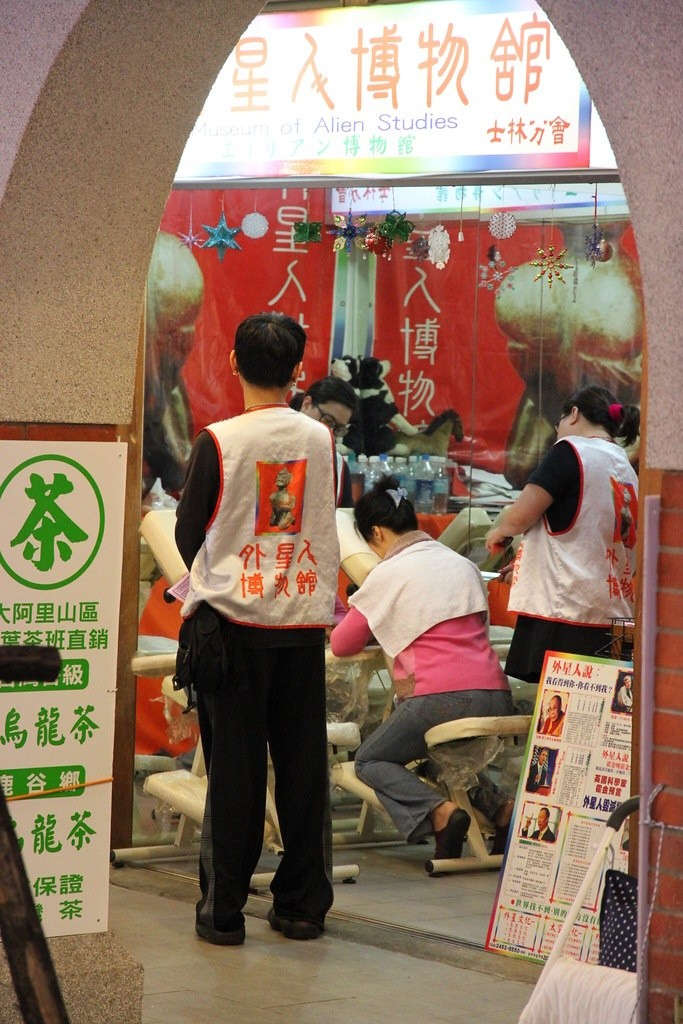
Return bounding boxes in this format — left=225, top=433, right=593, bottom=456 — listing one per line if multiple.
left=588, top=436, right=616, bottom=443
left=245, top=402, right=288, bottom=413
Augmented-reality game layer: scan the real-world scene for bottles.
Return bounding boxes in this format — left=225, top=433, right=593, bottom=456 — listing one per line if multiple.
left=347, top=450, right=450, bottom=516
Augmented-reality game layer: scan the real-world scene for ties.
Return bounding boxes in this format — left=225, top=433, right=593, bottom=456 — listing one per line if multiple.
left=539, top=767, right=542, bottom=775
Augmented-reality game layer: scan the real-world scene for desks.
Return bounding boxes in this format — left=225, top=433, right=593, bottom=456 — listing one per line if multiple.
left=132, top=623, right=517, bottom=834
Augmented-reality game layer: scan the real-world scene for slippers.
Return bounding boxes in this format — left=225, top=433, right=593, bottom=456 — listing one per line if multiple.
left=489, top=822, right=509, bottom=855
left=434, top=809, right=472, bottom=861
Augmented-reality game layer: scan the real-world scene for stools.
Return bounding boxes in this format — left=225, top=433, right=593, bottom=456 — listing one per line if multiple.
left=335, top=715, right=535, bottom=874
left=113, top=719, right=362, bottom=896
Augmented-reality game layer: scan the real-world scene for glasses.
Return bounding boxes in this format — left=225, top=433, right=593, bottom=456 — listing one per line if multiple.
left=547, top=706, right=559, bottom=713
left=315, top=405, right=349, bottom=438
left=555, top=412, right=571, bottom=433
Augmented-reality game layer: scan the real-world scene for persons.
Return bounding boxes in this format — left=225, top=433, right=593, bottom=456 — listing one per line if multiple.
left=522, top=808, right=555, bottom=842
left=617, top=676, right=632, bottom=711
left=482, top=383, right=640, bottom=684
left=291, top=376, right=353, bottom=508
left=179, top=311, right=339, bottom=947
left=540, top=696, right=564, bottom=734
left=526, top=747, right=549, bottom=793
left=333, top=472, right=514, bottom=860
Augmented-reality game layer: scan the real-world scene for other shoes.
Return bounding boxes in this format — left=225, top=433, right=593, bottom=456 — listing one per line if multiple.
left=194, top=920, right=246, bottom=946
left=268, top=907, right=324, bottom=939
